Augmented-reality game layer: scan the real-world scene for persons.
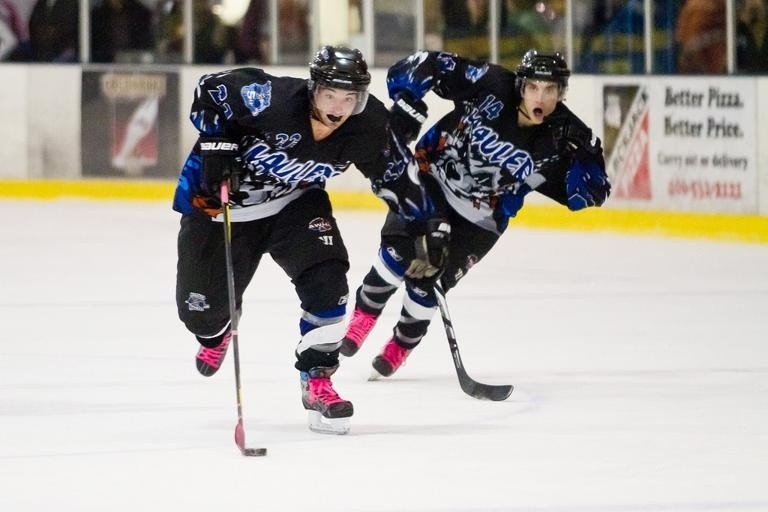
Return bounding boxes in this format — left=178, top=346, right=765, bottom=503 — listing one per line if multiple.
left=170, top=43, right=457, bottom=418
left=1, top=0, right=767, bottom=77
left=343, top=46, right=613, bottom=376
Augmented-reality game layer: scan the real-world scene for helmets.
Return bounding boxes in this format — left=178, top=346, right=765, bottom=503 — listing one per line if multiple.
left=513, top=49, right=571, bottom=102
left=310, top=45, right=372, bottom=116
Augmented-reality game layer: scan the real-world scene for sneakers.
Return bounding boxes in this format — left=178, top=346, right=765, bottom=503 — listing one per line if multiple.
left=196, top=331, right=231, bottom=377
left=372, top=337, right=411, bottom=377
left=338, top=308, right=376, bottom=357
left=300, top=364, right=352, bottom=419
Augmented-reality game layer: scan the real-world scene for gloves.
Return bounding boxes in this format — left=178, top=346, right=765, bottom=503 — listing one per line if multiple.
left=202, top=135, right=249, bottom=210
left=391, top=92, right=428, bottom=145
left=405, top=214, right=452, bottom=288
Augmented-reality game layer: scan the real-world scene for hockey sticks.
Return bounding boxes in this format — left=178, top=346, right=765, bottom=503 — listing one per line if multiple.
left=221, top=180, right=244, bottom=450
left=407, top=140, right=513, bottom=401
